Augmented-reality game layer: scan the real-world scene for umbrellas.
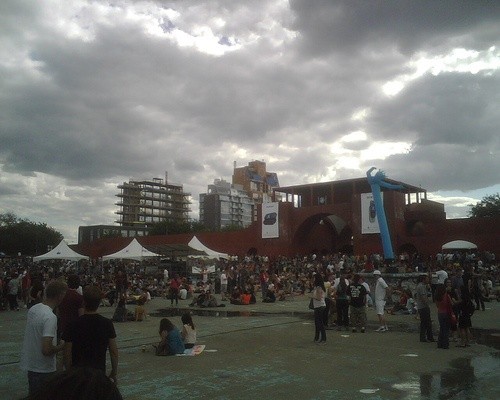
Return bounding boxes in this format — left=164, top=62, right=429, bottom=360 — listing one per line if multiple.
left=442, top=240, right=477, bottom=256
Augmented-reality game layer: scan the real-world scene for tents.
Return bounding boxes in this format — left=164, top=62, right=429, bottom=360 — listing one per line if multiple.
left=102, top=234, right=230, bottom=265
left=33, top=238, right=90, bottom=263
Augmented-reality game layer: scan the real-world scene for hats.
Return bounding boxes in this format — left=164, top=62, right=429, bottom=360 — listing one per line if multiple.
left=373, top=269, right=381, bottom=275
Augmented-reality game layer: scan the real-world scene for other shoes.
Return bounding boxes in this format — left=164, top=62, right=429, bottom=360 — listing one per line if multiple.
left=376, top=326, right=388, bottom=332
left=326, top=323, right=366, bottom=333
left=420, top=337, right=472, bottom=349
left=312, top=339, right=327, bottom=344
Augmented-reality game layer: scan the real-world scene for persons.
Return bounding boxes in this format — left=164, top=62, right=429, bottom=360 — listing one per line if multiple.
left=0, top=251, right=500, bottom=400
left=307, top=274, right=328, bottom=344
left=17, top=368, right=123, bottom=400
